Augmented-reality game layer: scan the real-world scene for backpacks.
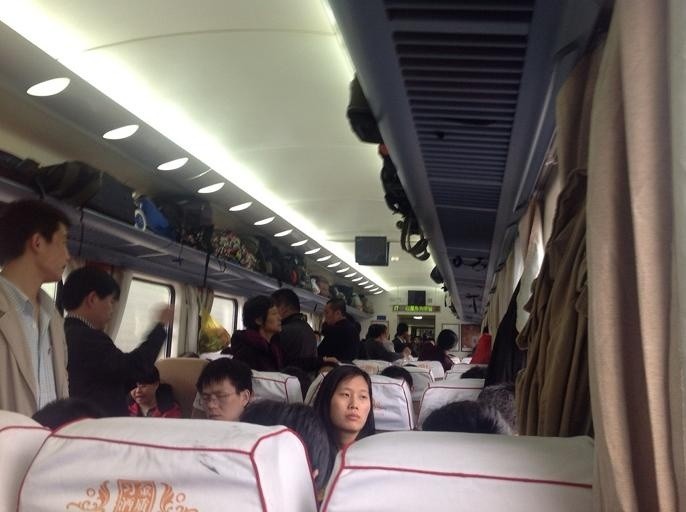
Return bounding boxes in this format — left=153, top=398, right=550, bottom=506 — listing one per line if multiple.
left=335, top=285, right=374, bottom=315
left=259, top=241, right=311, bottom=291
left=188, top=226, right=255, bottom=269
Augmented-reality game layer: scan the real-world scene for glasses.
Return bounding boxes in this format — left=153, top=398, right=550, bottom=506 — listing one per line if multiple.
left=196, top=390, right=239, bottom=407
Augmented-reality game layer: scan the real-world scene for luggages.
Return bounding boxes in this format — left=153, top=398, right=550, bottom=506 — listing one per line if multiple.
left=316, top=277, right=330, bottom=297
left=1, top=146, right=214, bottom=231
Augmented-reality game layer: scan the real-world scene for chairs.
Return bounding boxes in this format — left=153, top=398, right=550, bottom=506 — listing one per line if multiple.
left=0, top=346, right=602, bottom=512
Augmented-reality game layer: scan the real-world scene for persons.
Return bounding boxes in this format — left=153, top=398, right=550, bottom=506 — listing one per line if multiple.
left=421, top=326, right=517, bottom=436
left=196, top=286, right=459, bottom=512
left=2, top=197, right=182, bottom=431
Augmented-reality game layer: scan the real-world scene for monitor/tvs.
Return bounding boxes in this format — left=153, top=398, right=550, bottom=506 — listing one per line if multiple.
left=356, top=237, right=387, bottom=264
left=408, top=290, right=426, bottom=306
left=359, top=242, right=389, bottom=266
